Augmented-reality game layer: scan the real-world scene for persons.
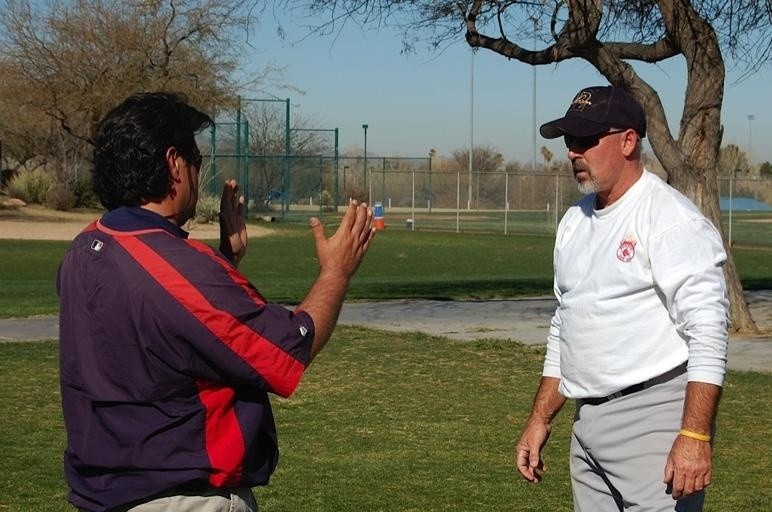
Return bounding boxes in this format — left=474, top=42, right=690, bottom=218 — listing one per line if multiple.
left=512, top=85, right=730, bottom=512
left=55, top=91, right=378, bottom=512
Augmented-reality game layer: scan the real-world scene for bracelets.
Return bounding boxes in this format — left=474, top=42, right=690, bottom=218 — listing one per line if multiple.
left=678, top=429, right=711, bottom=443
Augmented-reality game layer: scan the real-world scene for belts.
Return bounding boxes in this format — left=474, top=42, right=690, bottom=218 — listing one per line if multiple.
left=584, top=359, right=689, bottom=406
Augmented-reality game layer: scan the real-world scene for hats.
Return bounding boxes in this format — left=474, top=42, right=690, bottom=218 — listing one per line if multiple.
left=540, top=86, right=646, bottom=139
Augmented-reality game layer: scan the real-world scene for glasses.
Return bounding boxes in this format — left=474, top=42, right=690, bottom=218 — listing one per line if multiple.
left=179, top=153, right=203, bottom=173
left=565, top=129, right=626, bottom=148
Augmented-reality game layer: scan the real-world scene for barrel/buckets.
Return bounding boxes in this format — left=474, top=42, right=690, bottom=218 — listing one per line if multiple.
left=374, top=217, right=385, bottom=230
left=375, top=201, right=384, bottom=217
